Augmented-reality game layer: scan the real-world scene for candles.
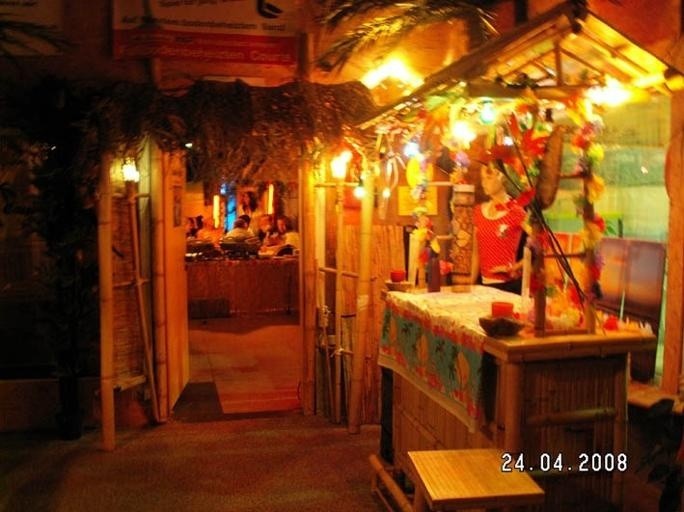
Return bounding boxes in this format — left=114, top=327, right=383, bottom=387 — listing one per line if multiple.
left=490, top=302, right=515, bottom=320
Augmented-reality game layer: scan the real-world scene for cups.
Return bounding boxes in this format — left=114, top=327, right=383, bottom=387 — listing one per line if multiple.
left=491, top=302, right=514, bottom=317
left=391, top=271, right=405, bottom=282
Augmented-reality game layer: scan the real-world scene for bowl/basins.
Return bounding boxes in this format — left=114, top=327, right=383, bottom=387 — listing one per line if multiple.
left=386, top=281, right=410, bottom=291
left=478, top=316, right=523, bottom=336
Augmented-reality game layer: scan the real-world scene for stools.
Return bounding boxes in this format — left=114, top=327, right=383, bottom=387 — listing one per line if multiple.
left=403, top=447, right=547, bottom=511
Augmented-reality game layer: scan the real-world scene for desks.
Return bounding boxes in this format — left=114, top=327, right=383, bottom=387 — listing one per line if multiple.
left=187, top=256, right=296, bottom=316
left=379, top=281, right=658, bottom=512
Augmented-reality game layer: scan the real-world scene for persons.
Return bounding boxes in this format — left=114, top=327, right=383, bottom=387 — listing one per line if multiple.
left=185, top=186, right=302, bottom=255
left=469, top=159, right=534, bottom=296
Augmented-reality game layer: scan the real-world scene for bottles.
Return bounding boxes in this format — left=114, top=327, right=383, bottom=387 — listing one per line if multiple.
left=428, top=250, right=441, bottom=292
